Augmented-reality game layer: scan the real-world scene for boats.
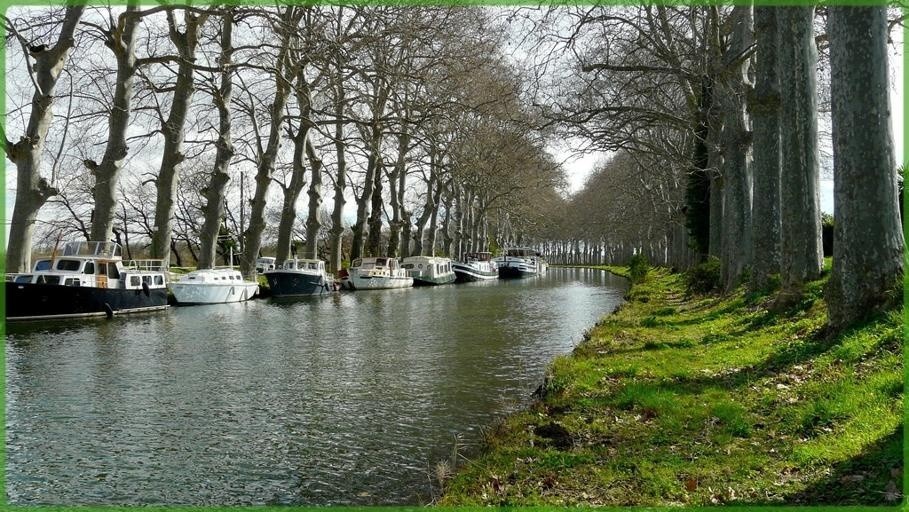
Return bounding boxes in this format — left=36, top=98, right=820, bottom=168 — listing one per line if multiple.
left=5, top=240, right=172, bottom=324
left=167, top=267, right=260, bottom=305
left=400, top=256, right=458, bottom=285
left=262, top=258, right=334, bottom=298
left=497, top=246, right=549, bottom=275
left=340, top=256, right=415, bottom=290
left=451, top=251, right=500, bottom=280
